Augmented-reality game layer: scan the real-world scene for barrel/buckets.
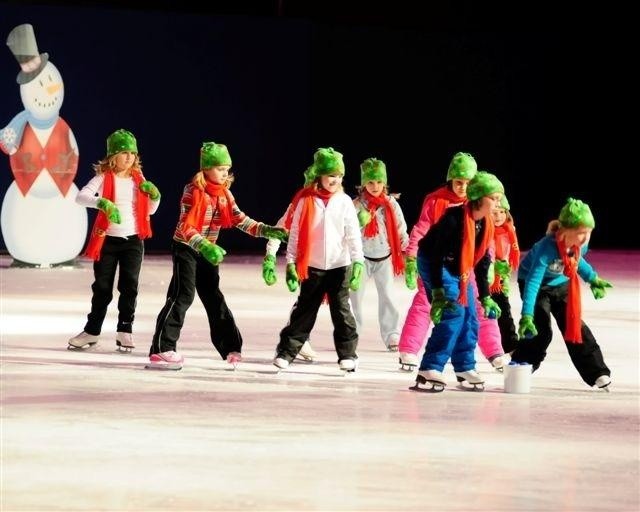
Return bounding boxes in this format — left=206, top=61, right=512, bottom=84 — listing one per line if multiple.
left=503, top=364, right=533, bottom=393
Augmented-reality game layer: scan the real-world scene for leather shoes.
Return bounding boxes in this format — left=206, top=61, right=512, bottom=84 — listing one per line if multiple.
left=339, top=357, right=359, bottom=369
left=272, top=354, right=290, bottom=369
left=115, top=331, right=135, bottom=348
left=68, top=331, right=99, bottom=348
left=596, top=375, right=612, bottom=389
left=386, top=334, right=418, bottom=368
left=416, top=368, right=447, bottom=386
left=299, top=338, right=318, bottom=357
left=493, top=352, right=511, bottom=370
left=454, top=369, right=486, bottom=385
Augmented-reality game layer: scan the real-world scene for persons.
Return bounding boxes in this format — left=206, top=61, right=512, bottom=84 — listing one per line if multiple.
left=398, top=151, right=512, bottom=368
left=273, top=147, right=364, bottom=370
left=416, top=172, right=505, bottom=383
left=262, top=164, right=344, bottom=358
left=489, top=195, right=520, bottom=356
left=510, top=197, right=615, bottom=388
left=149, top=141, right=288, bottom=363
left=351, top=157, right=409, bottom=347
left=68, top=128, right=162, bottom=348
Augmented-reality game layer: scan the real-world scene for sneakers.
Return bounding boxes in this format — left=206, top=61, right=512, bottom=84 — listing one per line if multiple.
left=150, top=350, right=183, bottom=363
left=226, top=351, right=243, bottom=363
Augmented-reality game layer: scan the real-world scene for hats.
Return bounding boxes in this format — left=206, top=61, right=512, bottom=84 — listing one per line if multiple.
left=200, top=140, right=232, bottom=170
left=360, top=156, right=388, bottom=186
left=467, top=170, right=511, bottom=211
left=105, top=127, right=139, bottom=156
left=302, top=146, right=346, bottom=183
left=558, top=197, right=596, bottom=228
left=446, top=152, right=479, bottom=184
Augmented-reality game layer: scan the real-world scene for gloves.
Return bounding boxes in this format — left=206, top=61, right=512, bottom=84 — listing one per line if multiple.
left=262, top=253, right=277, bottom=285
left=429, top=296, right=454, bottom=324
left=359, top=206, right=371, bottom=226
left=404, top=256, right=418, bottom=291
left=285, top=262, right=300, bottom=292
left=263, top=224, right=286, bottom=239
left=495, top=260, right=511, bottom=296
left=348, top=262, right=363, bottom=291
left=95, top=195, right=122, bottom=226
left=516, top=315, right=538, bottom=339
left=140, top=178, right=159, bottom=200
left=481, top=295, right=502, bottom=319
left=197, top=238, right=227, bottom=266
left=588, top=277, right=613, bottom=299
